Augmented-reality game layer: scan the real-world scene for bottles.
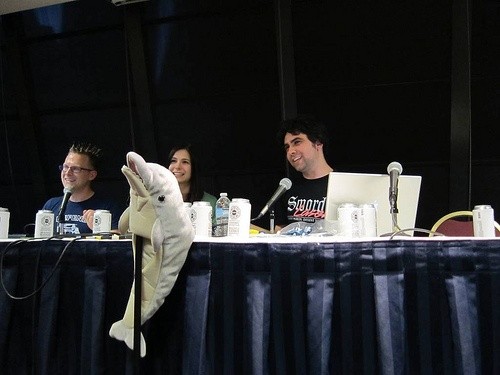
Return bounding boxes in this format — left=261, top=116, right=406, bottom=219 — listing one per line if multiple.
left=215, top=193, right=230, bottom=237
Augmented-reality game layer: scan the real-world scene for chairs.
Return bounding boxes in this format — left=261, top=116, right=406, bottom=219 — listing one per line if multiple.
left=428, top=210, right=500, bottom=238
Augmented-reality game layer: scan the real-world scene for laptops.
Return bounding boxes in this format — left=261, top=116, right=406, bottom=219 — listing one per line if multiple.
left=323, top=172, right=422, bottom=236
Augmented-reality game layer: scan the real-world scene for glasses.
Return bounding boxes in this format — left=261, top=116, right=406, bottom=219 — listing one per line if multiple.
left=60, top=164, right=94, bottom=173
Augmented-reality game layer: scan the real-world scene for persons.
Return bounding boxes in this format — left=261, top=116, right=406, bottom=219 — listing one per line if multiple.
left=39, top=142, right=126, bottom=238
left=157, top=143, right=219, bottom=234
left=267, top=118, right=347, bottom=234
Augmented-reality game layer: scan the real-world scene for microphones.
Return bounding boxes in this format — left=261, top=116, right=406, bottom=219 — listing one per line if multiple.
left=388, top=162, right=403, bottom=206
left=58, top=185, right=75, bottom=220
left=258, top=178, right=292, bottom=218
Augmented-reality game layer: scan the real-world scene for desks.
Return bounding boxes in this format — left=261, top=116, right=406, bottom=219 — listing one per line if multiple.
left=0, top=236, right=500, bottom=375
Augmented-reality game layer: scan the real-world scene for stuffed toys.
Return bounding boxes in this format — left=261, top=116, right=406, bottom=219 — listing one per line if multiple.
left=108, top=151, right=196, bottom=357
